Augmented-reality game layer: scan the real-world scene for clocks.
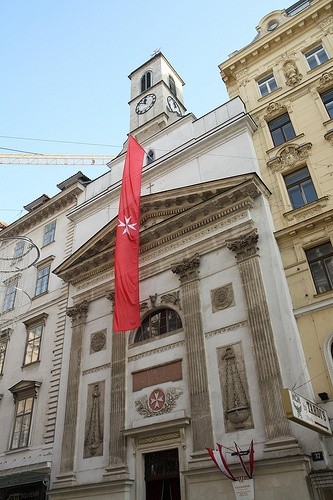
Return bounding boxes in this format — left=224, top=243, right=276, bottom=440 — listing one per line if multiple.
left=134, top=93, right=156, bottom=115
left=166, top=95, right=182, bottom=117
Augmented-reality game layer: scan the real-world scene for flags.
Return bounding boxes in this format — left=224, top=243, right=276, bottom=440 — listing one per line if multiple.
left=234, top=441, right=250, bottom=480
left=248, top=439, right=254, bottom=479
left=207, top=447, right=232, bottom=481
left=215, top=443, right=237, bottom=481
left=110, top=136, right=147, bottom=332
